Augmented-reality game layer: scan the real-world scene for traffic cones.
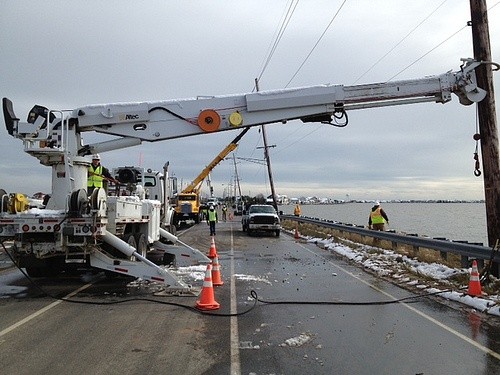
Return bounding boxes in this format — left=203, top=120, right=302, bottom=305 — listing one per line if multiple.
left=212, top=255, right=223, bottom=285
left=466, top=260, right=484, bottom=298
left=294, top=226, right=300, bottom=237
left=208, top=236, right=218, bottom=258
left=195, top=264, right=220, bottom=311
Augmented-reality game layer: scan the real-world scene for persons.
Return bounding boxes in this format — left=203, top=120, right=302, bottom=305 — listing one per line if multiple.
left=293, top=204, right=301, bottom=217
left=87, top=153, right=119, bottom=214
left=368, top=201, right=390, bottom=242
left=206, top=205, right=218, bottom=236
left=221, top=205, right=228, bottom=222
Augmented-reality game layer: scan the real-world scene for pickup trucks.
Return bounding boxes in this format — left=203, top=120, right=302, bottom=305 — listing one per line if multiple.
left=242, top=205, right=282, bottom=236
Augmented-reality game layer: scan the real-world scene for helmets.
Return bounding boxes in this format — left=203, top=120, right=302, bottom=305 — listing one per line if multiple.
left=223, top=205, right=225, bottom=207
left=92, top=153, right=100, bottom=159
left=374, top=201, right=380, bottom=205
left=210, top=206, right=214, bottom=209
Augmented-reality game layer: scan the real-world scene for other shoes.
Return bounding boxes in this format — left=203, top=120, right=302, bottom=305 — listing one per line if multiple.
left=214, top=232, right=216, bottom=235
left=210, top=233, right=212, bottom=236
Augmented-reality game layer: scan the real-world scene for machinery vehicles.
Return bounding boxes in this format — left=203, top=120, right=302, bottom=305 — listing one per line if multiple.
left=0, top=57, right=488, bottom=296
left=172, top=126, right=251, bottom=225
